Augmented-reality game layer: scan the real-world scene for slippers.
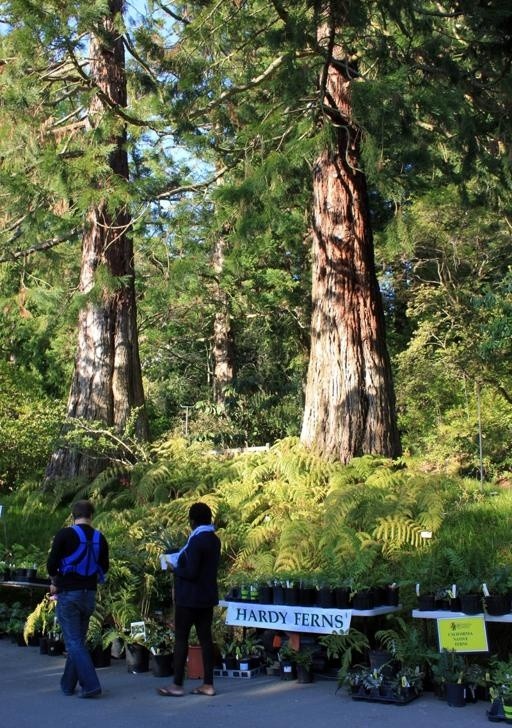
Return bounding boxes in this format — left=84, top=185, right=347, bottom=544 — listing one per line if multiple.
left=156, top=686, right=185, bottom=698
left=188, top=687, right=217, bottom=697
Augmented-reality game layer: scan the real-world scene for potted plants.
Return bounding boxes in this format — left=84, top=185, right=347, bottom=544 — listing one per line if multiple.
left=0, top=535, right=511, bottom=724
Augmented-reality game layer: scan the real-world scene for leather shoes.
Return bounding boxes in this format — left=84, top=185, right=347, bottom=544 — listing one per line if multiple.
left=78, top=685, right=102, bottom=699
left=60, top=674, right=77, bottom=696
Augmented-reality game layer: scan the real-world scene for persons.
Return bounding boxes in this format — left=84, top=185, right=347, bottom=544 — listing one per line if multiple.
left=156, top=502, right=221, bottom=697
left=46, top=501, right=108, bottom=699
